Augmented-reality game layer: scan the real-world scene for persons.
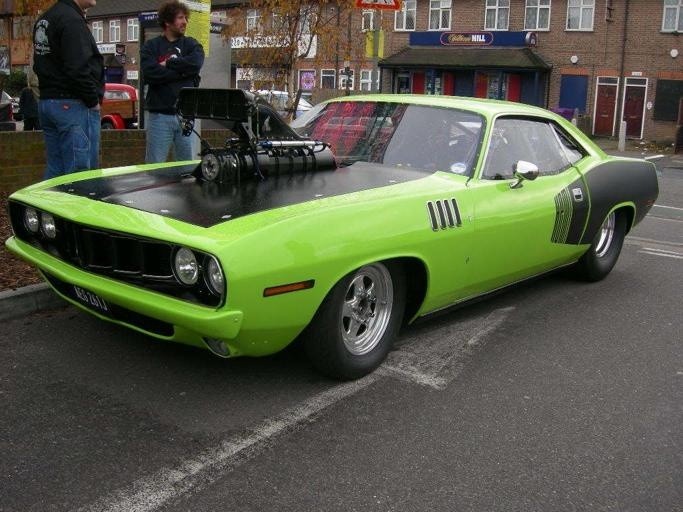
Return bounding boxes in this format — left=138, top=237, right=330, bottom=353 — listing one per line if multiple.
left=140, top=0, right=206, bottom=165
left=30, top=1, right=106, bottom=181
left=18, top=68, right=42, bottom=131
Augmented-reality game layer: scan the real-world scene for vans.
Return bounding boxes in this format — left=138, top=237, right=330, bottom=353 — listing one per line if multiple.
left=99, top=84, right=139, bottom=129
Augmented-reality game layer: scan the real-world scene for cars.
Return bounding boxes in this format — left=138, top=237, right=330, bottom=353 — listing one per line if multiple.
left=233, top=90, right=314, bottom=132
left=0, top=91, right=22, bottom=122
left=3, top=93, right=660, bottom=382
